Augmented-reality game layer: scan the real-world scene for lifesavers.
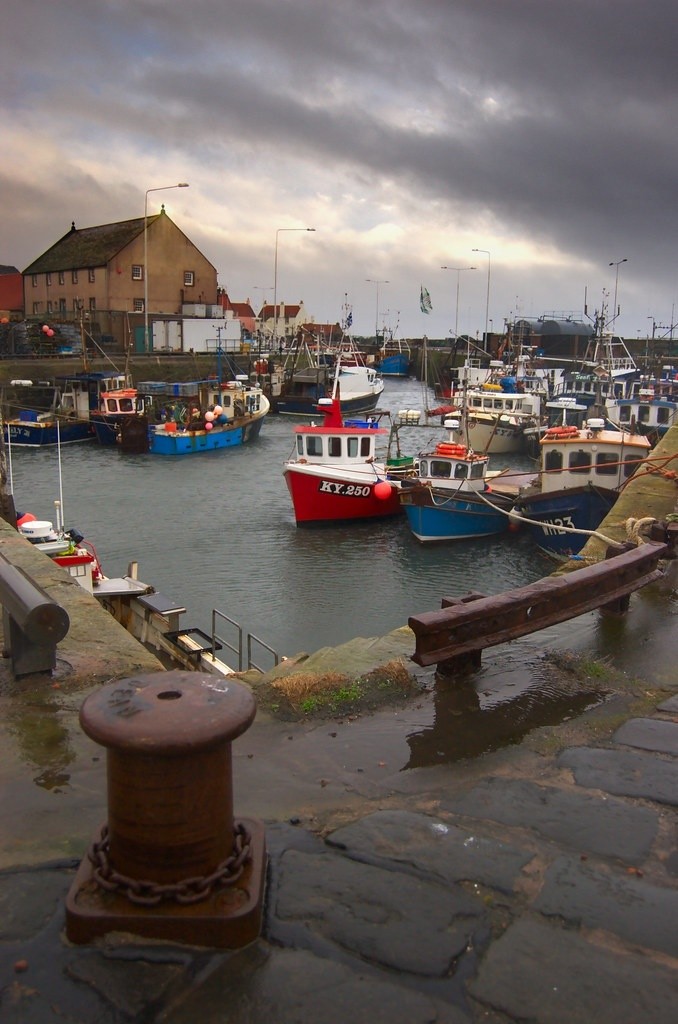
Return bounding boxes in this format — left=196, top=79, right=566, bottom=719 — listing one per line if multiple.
left=545, top=426, right=579, bottom=433
left=547, top=432, right=581, bottom=439
left=437, top=449, right=466, bottom=455
left=436, top=443, right=466, bottom=450
left=221, top=383, right=235, bottom=390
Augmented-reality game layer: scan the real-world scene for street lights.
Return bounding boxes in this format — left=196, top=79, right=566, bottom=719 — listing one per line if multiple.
left=472, top=249, right=491, bottom=352
left=274, top=227, right=316, bottom=348
left=365, top=279, right=389, bottom=344
left=609, top=259, right=628, bottom=343
left=144, top=183, right=189, bottom=355
left=441, top=266, right=476, bottom=348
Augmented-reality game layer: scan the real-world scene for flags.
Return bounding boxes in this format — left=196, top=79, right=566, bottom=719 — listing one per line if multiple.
left=420, top=287, right=433, bottom=315
left=346, top=311, right=353, bottom=328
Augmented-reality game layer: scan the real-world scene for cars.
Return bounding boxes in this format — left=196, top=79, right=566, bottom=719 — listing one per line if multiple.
left=85, top=335, right=128, bottom=358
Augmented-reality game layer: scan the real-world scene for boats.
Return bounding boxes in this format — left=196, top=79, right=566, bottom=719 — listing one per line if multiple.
left=4, top=293, right=411, bottom=454
left=282, top=287, right=678, bottom=543
left=8, top=420, right=278, bottom=675
